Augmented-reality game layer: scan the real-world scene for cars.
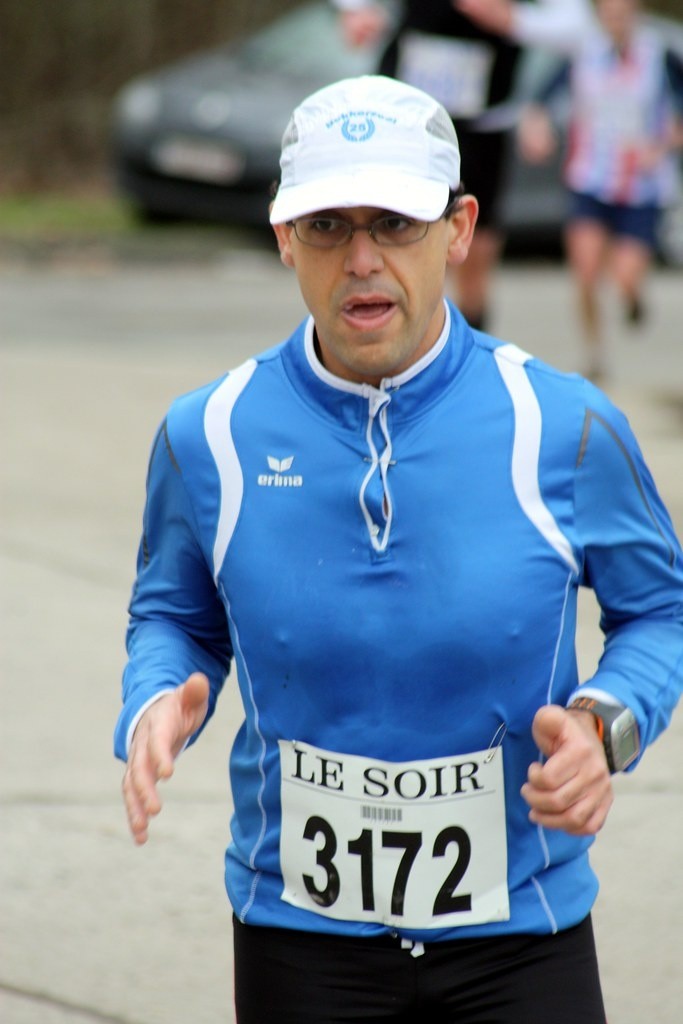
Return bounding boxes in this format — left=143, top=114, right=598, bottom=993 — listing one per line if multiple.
left=102, top=1, right=683, bottom=268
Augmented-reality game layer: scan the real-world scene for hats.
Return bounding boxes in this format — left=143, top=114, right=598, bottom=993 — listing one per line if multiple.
left=269, top=75, right=461, bottom=224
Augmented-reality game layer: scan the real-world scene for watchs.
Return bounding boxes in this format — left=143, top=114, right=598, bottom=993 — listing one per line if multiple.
left=567, top=698, right=641, bottom=774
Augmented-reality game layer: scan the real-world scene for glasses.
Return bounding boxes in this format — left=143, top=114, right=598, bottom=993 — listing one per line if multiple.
left=285, top=197, right=459, bottom=248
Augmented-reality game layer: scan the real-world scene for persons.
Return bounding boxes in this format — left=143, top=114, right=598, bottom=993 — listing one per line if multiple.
left=114, top=74, right=682, bottom=1024
left=330, top=0, right=582, bottom=330
left=523, top=1, right=677, bottom=384
